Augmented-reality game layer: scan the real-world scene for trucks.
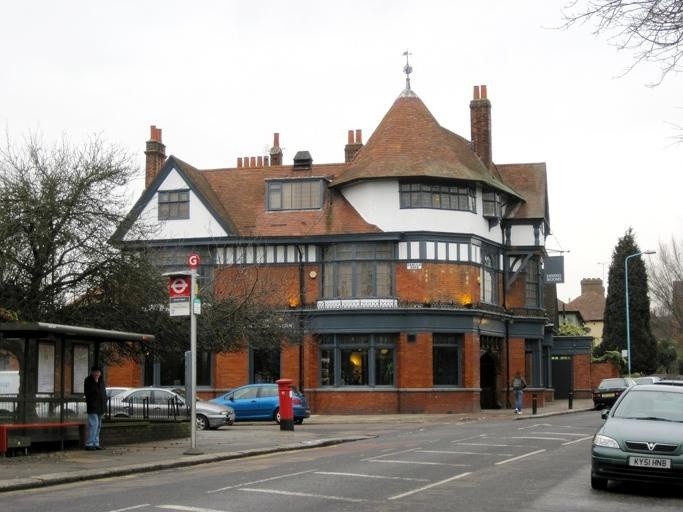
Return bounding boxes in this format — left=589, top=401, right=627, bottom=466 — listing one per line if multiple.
left=0, top=370, right=53, bottom=418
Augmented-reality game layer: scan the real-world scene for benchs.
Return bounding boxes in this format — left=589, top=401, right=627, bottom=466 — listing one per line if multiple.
left=0, top=422, right=87, bottom=459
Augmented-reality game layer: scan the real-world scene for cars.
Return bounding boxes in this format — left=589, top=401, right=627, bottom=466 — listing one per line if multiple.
left=654, top=373, right=683, bottom=386
left=54, top=384, right=134, bottom=419
left=592, top=377, right=637, bottom=409
left=105, top=386, right=237, bottom=431
left=631, top=375, right=660, bottom=387
left=589, top=384, right=682, bottom=495
left=207, top=381, right=311, bottom=427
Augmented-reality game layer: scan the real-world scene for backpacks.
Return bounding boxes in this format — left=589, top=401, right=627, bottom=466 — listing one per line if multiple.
left=513, top=378, right=521, bottom=390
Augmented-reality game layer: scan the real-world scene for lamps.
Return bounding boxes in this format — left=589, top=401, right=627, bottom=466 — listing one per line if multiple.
left=422, top=301, right=431, bottom=308
left=464, top=302, right=474, bottom=309
left=288, top=303, right=297, bottom=310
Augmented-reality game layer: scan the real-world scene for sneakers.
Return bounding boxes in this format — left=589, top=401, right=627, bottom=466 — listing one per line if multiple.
left=513, top=407, right=518, bottom=413
left=84, top=445, right=96, bottom=450
left=95, top=446, right=106, bottom=450
left=518, top=411, right=522, bottom=415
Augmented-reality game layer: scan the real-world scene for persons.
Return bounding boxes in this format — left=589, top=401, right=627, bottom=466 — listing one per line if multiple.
left=511, top=372, right=527, bottom=414
left=83, top=366, right=108, bottom=451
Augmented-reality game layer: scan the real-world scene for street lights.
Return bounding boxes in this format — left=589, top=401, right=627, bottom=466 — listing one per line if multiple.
left=625, top=245, right=657, bottom=379
